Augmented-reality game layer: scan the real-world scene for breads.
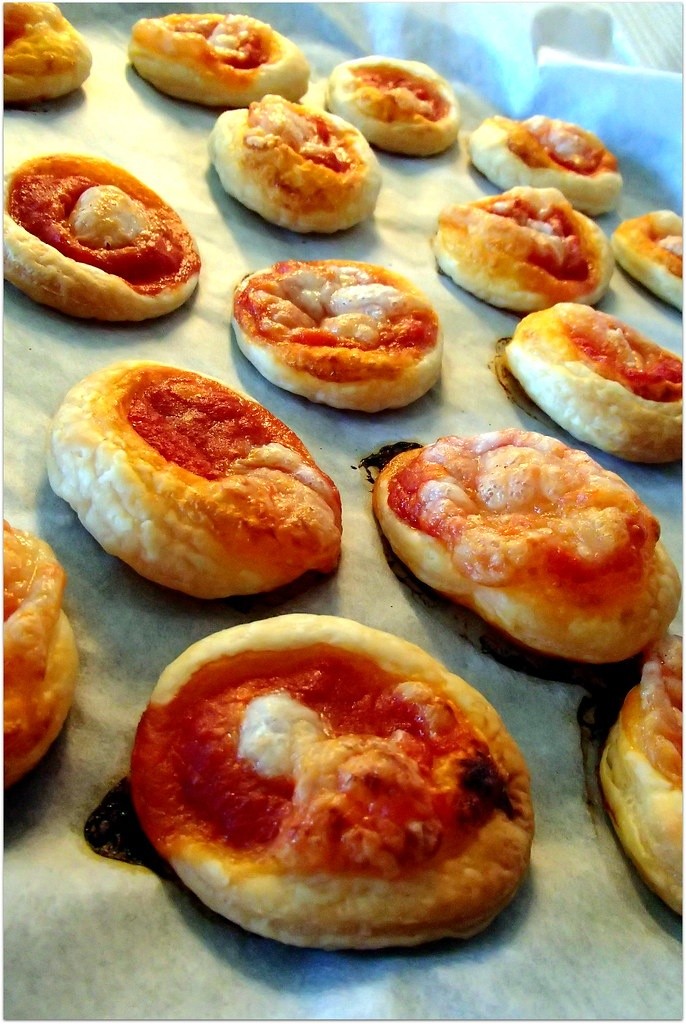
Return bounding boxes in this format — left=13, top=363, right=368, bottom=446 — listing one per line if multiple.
left=5, top=1, right=683, bottom=956
